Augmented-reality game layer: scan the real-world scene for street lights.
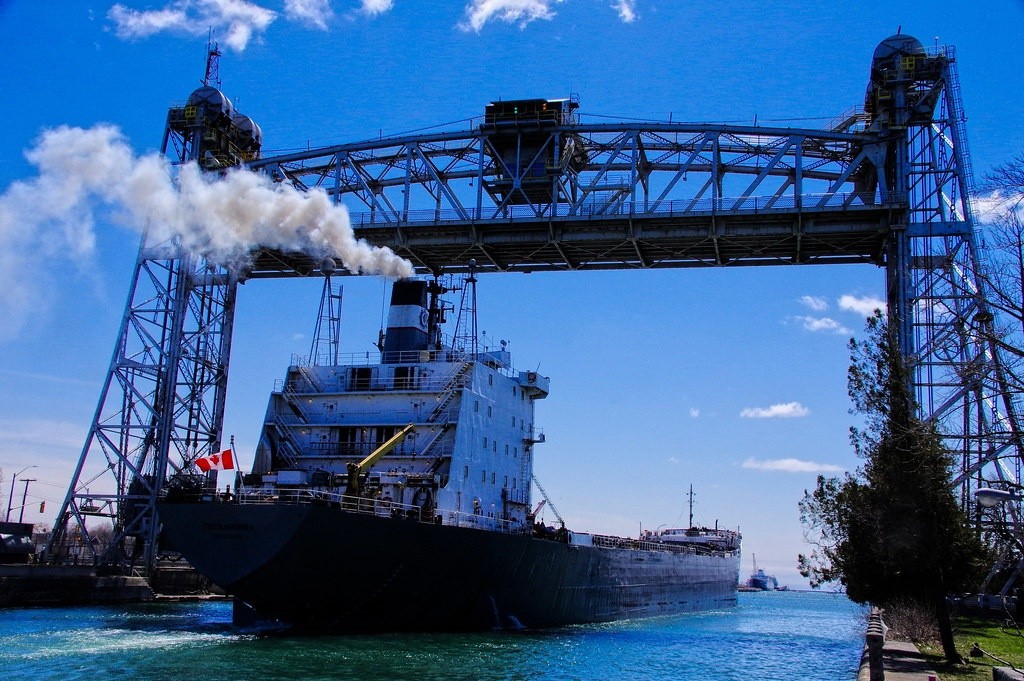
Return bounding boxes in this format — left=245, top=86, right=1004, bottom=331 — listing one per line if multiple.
left=6, top=464, right=39, bottom=523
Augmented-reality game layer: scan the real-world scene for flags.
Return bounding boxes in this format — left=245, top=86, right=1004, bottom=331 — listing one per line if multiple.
left=194, top=449, right=234, bottom=473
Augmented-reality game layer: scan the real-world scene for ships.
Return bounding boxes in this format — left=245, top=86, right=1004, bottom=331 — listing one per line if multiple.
left=157, top=258, right=741, bottom=639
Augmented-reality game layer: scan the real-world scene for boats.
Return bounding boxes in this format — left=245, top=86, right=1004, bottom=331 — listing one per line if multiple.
left=738, top=568, right=789, bottom=592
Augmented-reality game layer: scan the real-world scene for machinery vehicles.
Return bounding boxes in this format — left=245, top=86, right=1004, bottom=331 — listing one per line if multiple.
left=341, top=421, right=417, bottom=514
